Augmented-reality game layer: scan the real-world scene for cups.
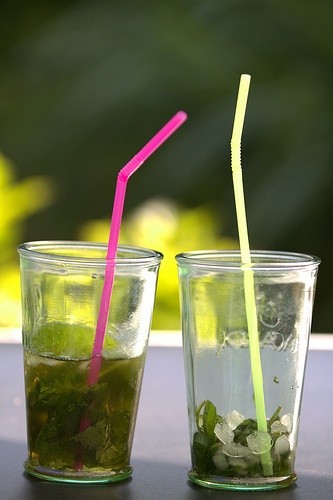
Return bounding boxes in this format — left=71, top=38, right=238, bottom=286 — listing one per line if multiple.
left=19, top=241, right=163, bottom=484
left=174, top=247, right=321, bottom=492
